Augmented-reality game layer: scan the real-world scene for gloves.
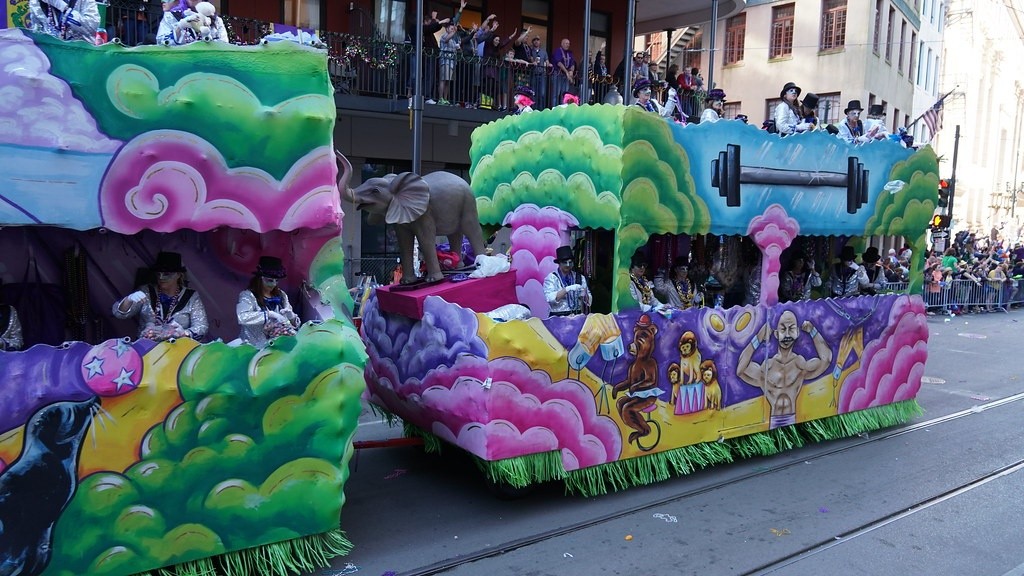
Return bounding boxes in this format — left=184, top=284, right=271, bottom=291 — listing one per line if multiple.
left=796, top=119, right=811, bottom=131
left=269, top=311, right=285, bottom=322
left=806, top=260, right=815, bottom=269
left=657, top=267, right=665, bottom=273
left=857, top=133, right=870, bottom=142
left=280, top=308, right=296, bottom=320
left=792, top=281, right=801, bottom=291
left=566, top=283, right=586, bottom=296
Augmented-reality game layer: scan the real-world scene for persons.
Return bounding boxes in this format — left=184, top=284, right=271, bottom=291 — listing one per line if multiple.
left=654, top=256, right=697, bottom=306
left=29, top=0, right=101, bottom=44
left=774, top=82, right=819, bottom=136
left=236, top=256, right=301, bottom=346
left=423, top=0, right=704, bottom=124
left=877, top=228, right=1024, bottom=315
left=836, top=100, right=887, bottom=143
left=631, top=252, right=670, bottom=311
left=543, top=245, right=592, bottom=317
left=700, top=89, right=727, bottom=122
left=723, top=246, right=884, bottom=310
left=0, top=277, right=24, bottom=351
left=112, top=252, right=209, bottom=341
left=107, top=0, right=229, bottom=45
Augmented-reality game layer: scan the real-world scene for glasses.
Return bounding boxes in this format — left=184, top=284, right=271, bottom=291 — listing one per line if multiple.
left=637, top=57, right=643, bottom=59
left=634, top=263, right=648, bottom=268
left=532, top=38, right=540, bottom=41
left=649, top=64, right=657, bottom=66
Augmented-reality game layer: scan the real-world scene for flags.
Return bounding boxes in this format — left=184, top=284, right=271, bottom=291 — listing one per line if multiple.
left=921, top=99, right=943, bottom=141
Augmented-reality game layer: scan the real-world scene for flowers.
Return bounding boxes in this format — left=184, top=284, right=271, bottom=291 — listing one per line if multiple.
left=222, top=14, right=401, bottom=71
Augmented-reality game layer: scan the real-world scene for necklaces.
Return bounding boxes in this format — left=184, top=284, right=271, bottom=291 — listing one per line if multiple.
left=631, top=273, right=651, bottom=304
left=675, top=277, right=692, bottom=303
left=154, top=284, right=180, bottom=324
left=558, top=268, right=575, bottom=309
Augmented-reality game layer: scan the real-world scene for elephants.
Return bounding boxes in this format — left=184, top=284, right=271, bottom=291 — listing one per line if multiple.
left=335, top=149, right=488, bottom=283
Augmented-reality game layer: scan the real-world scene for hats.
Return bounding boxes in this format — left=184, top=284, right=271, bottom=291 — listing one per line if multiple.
left=781, top=83, right=801, bottom=100
left=633, top=78, right=656, bottom=98
left=554, top=246, right=574, bottom=263
left=959, top=260, right=967, bottom=265
left=789, top=251, right=806, bottom=262
left=253, top=256, right=287, bottom=278
left=631, top=246, right=653, bottom=261
left=800, top=94, right=819, bottom=109
left=151, top=252, right=186, bottom=273
left=705, top=89, right=727, bottom=103
left=869, top=105, right=886, bottom=116
left=889, top=248, right=895, bottom=254
left=513, top=85, right=535, bottom=101
left=838, top=246, right=857, bottom=260
left=844, top=101, right=863, bottom=115
left=673, top=256, right=690, bottom=266
left=563, top=86, right=579, bottom=96
left=862, top=247, right=880, bottom=263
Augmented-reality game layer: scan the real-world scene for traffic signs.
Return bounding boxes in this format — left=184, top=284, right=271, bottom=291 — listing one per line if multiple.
left=931, top=229, right=952, bottom=240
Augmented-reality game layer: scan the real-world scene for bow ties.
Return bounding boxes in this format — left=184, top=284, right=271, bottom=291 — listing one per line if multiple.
left=160, top=293, right=174, bottom=304
left=805, top=117, right=814, bottom=123
left=263, top=296, right=281, bottom=304
left=853, top=126, right=861, bottom=133
left=841, top=268, right=851, bottom=273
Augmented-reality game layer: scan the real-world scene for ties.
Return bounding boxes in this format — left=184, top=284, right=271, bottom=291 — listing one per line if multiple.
left=639, top=279, right=645, bottom=287
left=681, top=281, right=688, bottom=292
left=646, top=103, right=653, bottom=112
left=565, top=276, right=570, bottom=286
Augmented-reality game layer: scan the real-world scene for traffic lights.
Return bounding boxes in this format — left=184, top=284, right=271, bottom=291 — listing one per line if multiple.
left=934, top=214, right=952, bottom=229
left=937, top=178, right=953, bottom=208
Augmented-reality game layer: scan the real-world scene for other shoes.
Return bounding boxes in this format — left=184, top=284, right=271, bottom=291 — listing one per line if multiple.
left=424, top=97, right=508, bottom=112
